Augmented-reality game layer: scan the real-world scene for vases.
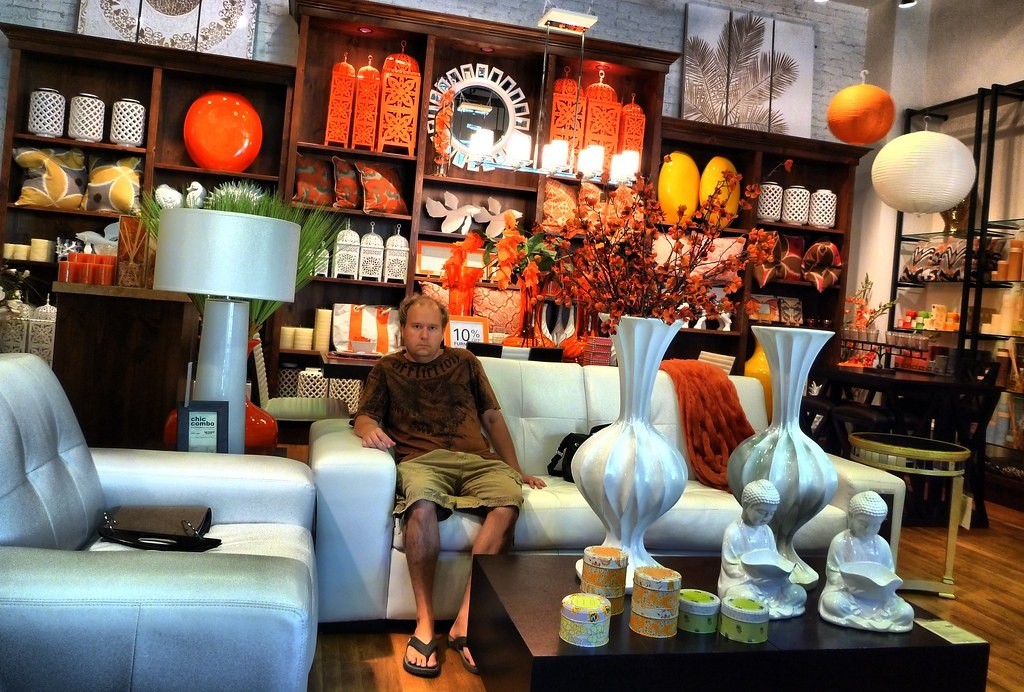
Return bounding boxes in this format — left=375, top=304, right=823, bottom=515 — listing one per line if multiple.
left=570, top=311, right=688, bottom=595
left=727, top=326, right=839, bottom=584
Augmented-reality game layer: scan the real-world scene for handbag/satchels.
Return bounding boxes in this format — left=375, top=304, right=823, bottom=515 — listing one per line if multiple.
left=546, top=424, right=612, bottom=484
left=99, top=503, right=221, bottom=552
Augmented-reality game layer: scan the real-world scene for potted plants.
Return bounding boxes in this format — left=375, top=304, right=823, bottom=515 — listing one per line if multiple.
left=132, top=184, right=352, bottom=454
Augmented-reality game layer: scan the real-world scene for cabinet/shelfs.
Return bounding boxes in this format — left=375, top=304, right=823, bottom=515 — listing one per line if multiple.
left=0, top=0, right=292, bottom=437
left=619, top=115, right=875, bottom=463
left=284, top=0, right=688, bottom=443
left=885, top=80, right=1024, bottom=511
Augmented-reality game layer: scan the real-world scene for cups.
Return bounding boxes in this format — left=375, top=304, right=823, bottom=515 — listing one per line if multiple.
left=885, top=330, right=928, bottom=350
left=842, top=328, right=879, bottom=342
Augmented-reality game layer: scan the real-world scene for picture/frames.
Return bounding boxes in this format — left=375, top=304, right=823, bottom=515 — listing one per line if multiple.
left=177, top=401, right=228, bottom=454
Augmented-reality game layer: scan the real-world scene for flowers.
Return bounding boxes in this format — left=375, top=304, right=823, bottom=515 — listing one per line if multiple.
left=844, top=273, right=898, bottom=328
left=440, top=156, right=792, bottom=336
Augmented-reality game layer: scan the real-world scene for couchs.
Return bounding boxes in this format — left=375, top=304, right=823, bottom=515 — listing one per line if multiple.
left=309, top=355, right=906, bottom=621
left=0, top=353, right=318, bottom=692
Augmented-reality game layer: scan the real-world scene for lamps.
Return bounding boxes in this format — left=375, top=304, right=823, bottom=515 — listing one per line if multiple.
left=467, top=9, right=640, bottom=185
left=152, top=207, right=302, bottom=455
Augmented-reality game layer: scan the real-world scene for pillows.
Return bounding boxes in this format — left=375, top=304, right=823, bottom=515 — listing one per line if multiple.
left=10, top=147, right=408, bottom=213
left=754, top=231, right=843, bottom=291
left=899, top=236, right=1007, bottom=282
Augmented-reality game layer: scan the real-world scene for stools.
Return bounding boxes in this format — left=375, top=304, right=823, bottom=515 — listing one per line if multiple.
left=800, top=395, right=898, bottom=460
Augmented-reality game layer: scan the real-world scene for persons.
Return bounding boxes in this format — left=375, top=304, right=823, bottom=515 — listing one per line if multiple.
left=349, top=293, right=548, bottom=677
left=716, top=478, right=807, bottom=619
left=818, top=491, right=914, bottom=633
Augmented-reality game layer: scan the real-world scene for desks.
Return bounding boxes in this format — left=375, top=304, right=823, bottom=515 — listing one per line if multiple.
left=467, top=554, right=989, bottom=692
left=847, top=431, right=970, bottom=598
left=839, top=366, right=1006, bottom=530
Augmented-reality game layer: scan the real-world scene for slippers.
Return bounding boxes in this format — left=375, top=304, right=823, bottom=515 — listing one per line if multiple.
left=447, top=633, right=480, bottom=675
left=402, top=634, right=441, bottom=677
